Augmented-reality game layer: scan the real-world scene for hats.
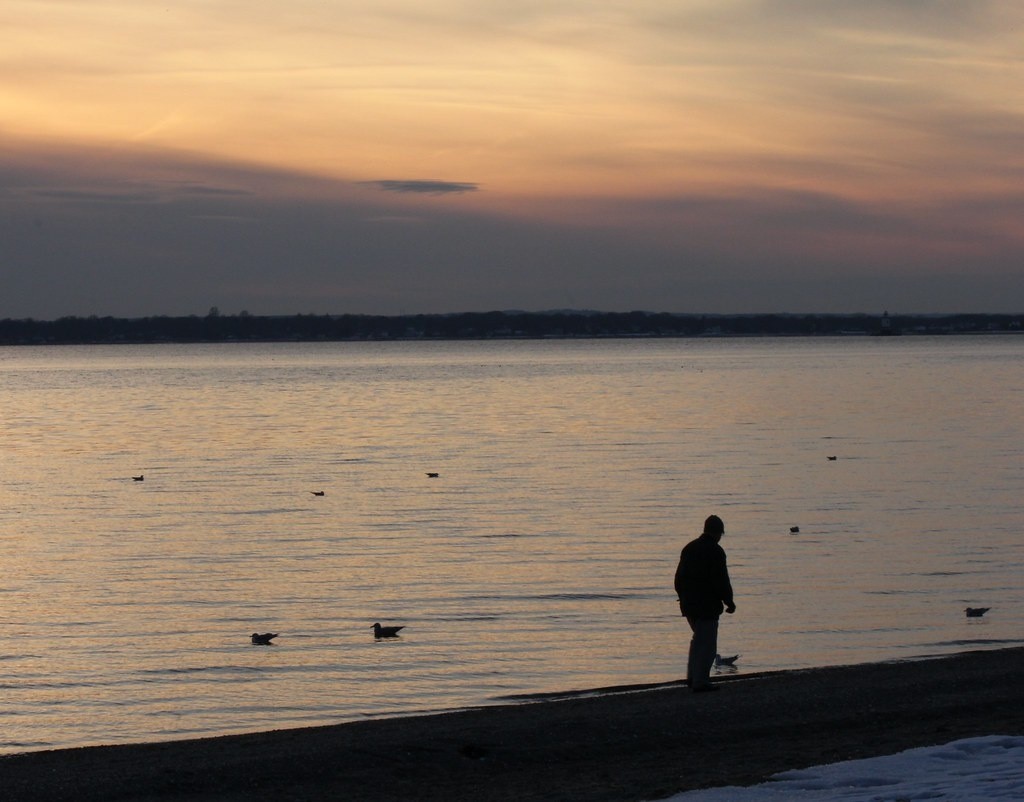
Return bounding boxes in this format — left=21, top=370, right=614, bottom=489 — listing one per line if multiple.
left=703, top=515, right=724, bottom=533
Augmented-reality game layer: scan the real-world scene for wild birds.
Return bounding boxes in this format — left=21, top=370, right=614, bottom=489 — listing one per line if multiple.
left=826, top=456, right=837, bottom=460
left=371, top=622, right=407, bottom=636
left=790, top=526, right=800, bottom=532
left=424, top=473, right=439, bottom=475
left=249, top=632, right=279, bottom=643
left=964, top=606, right=992, bottom=617
left=309, top=490, right=324, bottom=496
left=132, top=475, right=145, bottom=480
left=714, top=654, right=741, bottom=665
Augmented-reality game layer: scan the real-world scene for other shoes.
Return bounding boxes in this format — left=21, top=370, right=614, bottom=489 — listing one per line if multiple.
left=689, top=683, right=720, bottom=691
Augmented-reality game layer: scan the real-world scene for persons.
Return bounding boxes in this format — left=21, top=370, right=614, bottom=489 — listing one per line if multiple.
left=674, top=516, right=736, bottom=691
